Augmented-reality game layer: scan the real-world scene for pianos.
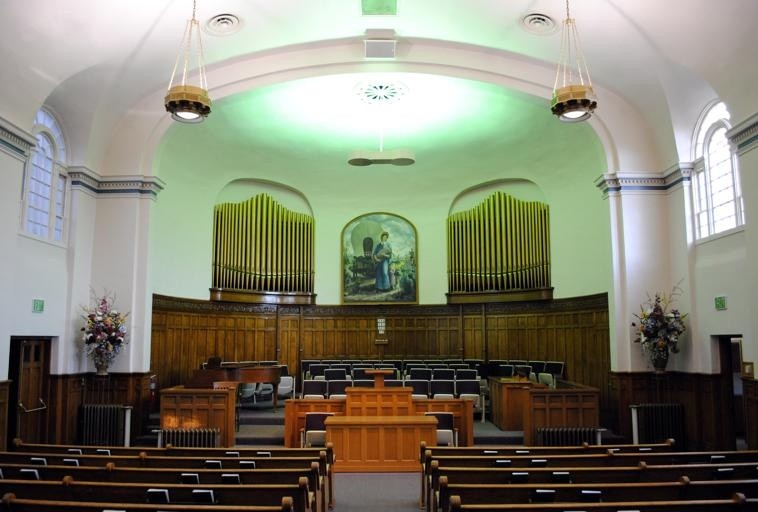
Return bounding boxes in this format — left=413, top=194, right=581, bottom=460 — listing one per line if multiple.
left=185, top=356, right=289, bottom=413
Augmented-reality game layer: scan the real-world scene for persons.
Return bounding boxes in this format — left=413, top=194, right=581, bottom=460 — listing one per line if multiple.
left=372, top=230, right=392, bottom=292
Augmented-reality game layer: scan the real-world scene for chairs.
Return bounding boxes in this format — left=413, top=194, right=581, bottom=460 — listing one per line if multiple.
left=199, top=354, right=564, bottom=447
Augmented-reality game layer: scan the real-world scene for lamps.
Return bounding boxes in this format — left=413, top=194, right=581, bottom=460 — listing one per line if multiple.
left=551, top=0, right=597, bottom=123
left=165, top=0, right=213, bottom=125
left=207, top=14, right=246, bottom=38
left=524, top=12, right=556, bottom=35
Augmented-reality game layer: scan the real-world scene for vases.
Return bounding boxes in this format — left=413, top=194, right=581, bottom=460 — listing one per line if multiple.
left=94, top=356, right=107, bottom=376
left=651, top=345, right=669, bottom=373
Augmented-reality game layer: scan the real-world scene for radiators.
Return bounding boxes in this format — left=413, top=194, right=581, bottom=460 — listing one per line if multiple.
left=161, top=427, right=221, bottom=448
left=83, top=402, right=123, bottom=447
left=536, top=426, right=597, bottom=447
left=639, top=401, right=687, bottom=444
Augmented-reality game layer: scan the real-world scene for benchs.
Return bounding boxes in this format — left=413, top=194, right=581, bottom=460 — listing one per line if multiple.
left=419, top=441, right=758, bottom=512
left=1, top=439, right=338, bottom=511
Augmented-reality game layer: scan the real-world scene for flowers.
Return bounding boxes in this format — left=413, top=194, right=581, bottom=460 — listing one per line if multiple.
left=628, top=272, right=688, bottom=355
left=75, top=283, right=130, bottom=362
